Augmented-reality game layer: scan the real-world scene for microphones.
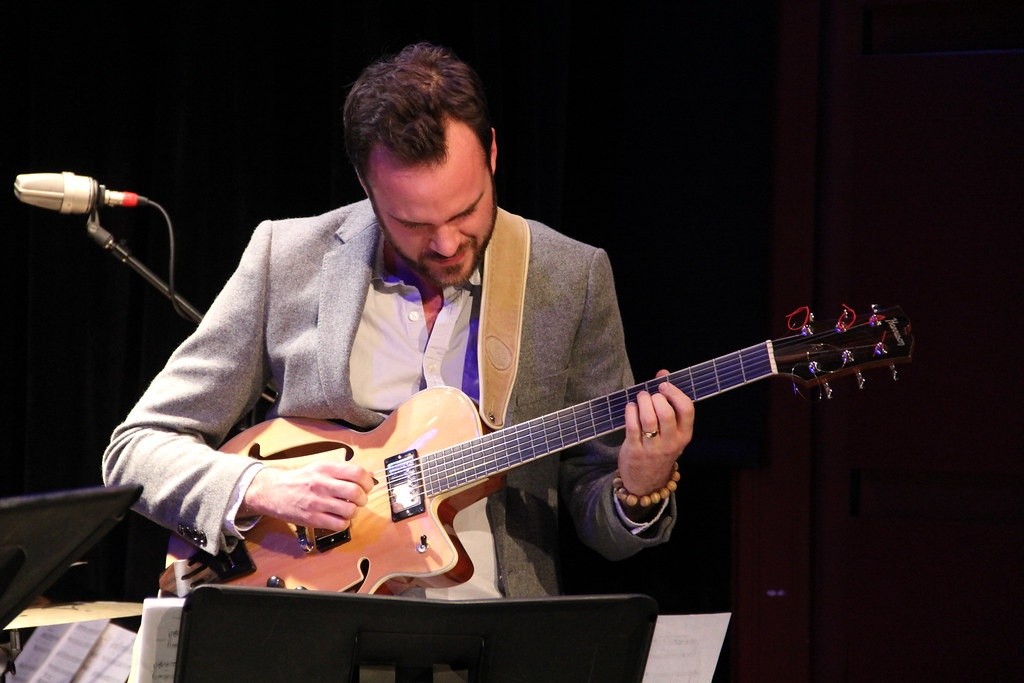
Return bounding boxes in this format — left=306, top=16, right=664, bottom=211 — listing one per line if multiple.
left=14, top=171, right=150, bottom=215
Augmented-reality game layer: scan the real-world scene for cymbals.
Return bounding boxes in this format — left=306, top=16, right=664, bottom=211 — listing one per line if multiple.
left=3, top=597, right=144, bottom=631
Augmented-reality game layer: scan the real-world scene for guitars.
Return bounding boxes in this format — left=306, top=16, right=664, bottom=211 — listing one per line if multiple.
left=157, top=300, right=918, bottom=599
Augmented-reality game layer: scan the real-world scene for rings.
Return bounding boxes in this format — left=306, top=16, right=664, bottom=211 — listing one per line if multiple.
left=642, top=428, right=660, bottom=440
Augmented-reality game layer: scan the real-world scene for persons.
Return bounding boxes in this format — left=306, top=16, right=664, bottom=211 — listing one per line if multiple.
left=100, top=41, right=698, bottom=608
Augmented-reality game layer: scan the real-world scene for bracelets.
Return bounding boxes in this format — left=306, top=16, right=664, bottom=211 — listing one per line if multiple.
left=612, top=462, right=680, bottom=507
